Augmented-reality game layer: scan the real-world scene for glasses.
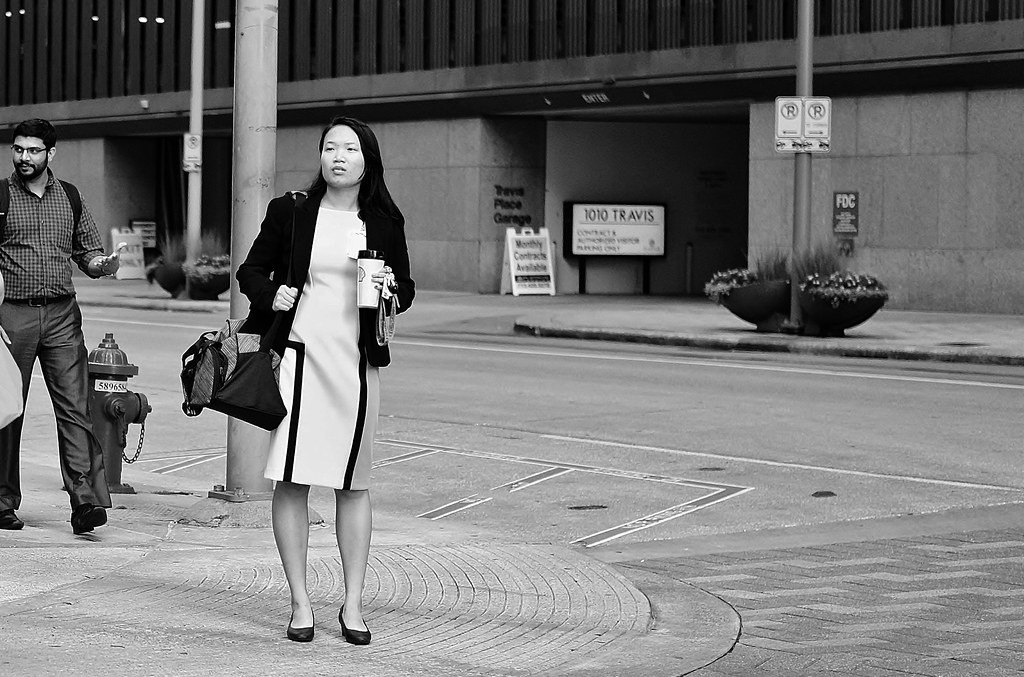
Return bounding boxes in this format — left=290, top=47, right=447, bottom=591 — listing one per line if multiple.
left=9, top=144, right=50, bottom=155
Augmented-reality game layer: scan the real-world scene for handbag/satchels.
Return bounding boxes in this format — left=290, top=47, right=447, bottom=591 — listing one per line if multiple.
left=180, top=318, right=287, bottom=432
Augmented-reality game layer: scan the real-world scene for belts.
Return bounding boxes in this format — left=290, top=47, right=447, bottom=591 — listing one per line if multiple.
left=3, top=296, right=76, bottom=307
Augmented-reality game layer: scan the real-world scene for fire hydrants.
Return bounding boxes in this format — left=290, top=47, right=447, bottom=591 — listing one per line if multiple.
left=88, top=333, right=153, bottom=495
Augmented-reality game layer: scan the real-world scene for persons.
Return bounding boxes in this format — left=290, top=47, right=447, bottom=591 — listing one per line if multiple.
left=0, top=118, right=127, bottom=534
left=236, top=119, right=415, bottom=644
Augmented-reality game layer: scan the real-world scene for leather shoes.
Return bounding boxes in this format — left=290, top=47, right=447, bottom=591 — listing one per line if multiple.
left=0, top=509, right=24, bottom=530
left=70, top=503, right=107, bottom=534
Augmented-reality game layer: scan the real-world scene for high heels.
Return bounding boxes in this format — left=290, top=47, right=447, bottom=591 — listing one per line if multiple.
left=287, top=608, right=315, bottom=642
left=338, top=604, right=372, bottom=645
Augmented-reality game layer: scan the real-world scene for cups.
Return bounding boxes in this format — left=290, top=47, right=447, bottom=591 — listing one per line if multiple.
left=356, top=250, right=386, bottom=309
